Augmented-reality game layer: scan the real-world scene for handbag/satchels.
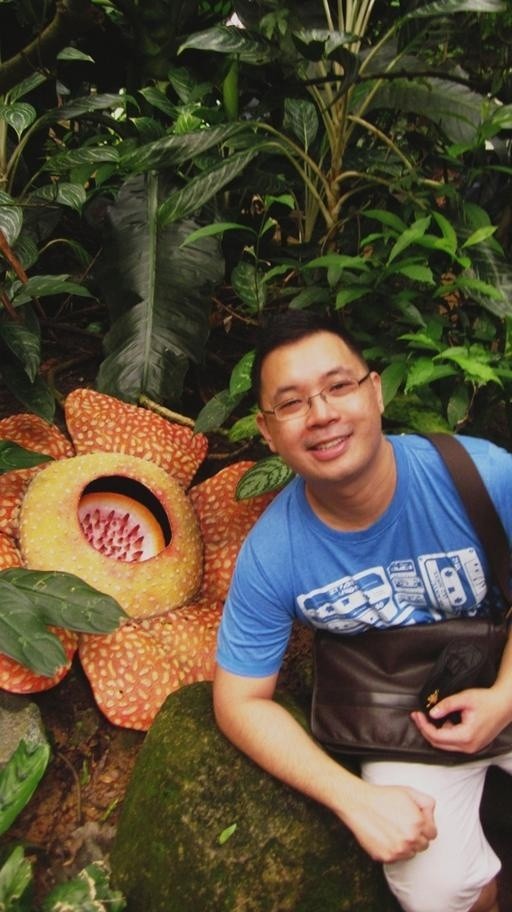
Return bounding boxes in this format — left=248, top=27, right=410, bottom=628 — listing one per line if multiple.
left=308, top=611, right=512, bottom=767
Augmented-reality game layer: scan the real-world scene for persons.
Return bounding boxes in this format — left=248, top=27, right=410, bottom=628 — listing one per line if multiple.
left=213, top=317, right=512, bottom=912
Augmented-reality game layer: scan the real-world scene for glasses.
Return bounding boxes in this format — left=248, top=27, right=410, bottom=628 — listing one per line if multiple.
left=263, top=374, right=373, bottom=422
